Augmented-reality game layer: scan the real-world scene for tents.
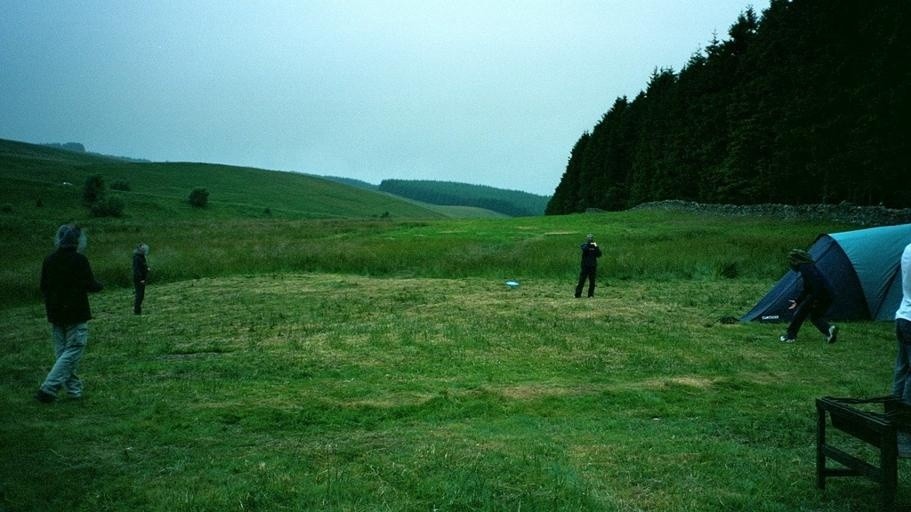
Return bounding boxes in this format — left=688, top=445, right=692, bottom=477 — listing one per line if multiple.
left=738, top=222, right=910, bottom=323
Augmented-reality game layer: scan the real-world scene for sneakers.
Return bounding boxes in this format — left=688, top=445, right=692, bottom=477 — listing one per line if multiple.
left=826, top=325, right=838, bottom=343
left=780, top=335, right=796, bottom=343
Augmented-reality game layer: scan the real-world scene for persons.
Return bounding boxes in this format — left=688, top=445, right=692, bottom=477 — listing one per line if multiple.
left=778, top=246, right=840, bottom=346
left=132, top=243, right=151, bottom=315
left=894, top=242, right=911, bottom=462
left=573, top=231, right=603, bottom=300
left=35, top=220, right=104, bottom=403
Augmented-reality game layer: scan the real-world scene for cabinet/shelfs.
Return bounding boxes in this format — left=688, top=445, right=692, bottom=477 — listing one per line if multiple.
left=810, top=395, right=910, bottom=510
